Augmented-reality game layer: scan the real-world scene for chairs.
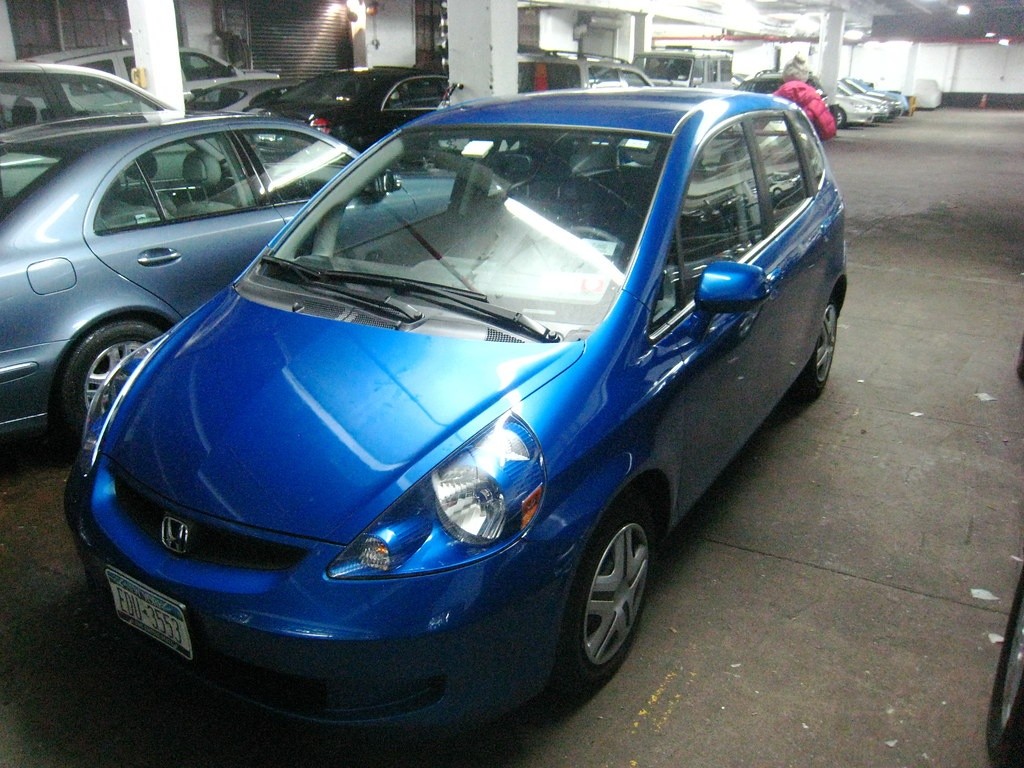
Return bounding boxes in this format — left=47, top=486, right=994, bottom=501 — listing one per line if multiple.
left=122, top=154, right=178, bottom=214
left=447, top=150, right=545, bottom=262
left=614, top=151, right=728, bottom=248
left=11, top=97, right=37, bottom=127
left=179, top=152, right=239, bottom=217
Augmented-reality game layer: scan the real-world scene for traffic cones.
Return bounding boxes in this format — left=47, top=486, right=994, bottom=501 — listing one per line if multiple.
left=978, top=93, right=988, bottom=108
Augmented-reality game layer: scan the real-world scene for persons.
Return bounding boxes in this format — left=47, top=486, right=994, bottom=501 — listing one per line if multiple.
left=773, top=54, right=837, bottom=141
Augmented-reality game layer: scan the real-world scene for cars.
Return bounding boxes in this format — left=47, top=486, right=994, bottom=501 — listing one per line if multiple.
left=61, top=88, right=853, bottom=734
left=0, top=41, right=451, bottom=183
left=980, top=336, right=1024, bottom=768
left=514, top=42, right=910, bottom=131
left=0, top=108, right=460, bottom=459
